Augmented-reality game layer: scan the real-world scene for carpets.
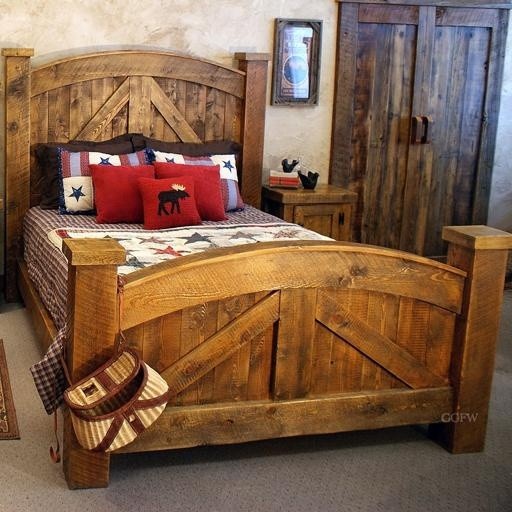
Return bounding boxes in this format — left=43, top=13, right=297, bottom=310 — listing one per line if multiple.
left=1, top=339, right=21, bottom=440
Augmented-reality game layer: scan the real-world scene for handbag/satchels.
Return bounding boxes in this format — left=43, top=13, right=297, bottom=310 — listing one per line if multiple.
left=63, top=346, right=169, bottom=453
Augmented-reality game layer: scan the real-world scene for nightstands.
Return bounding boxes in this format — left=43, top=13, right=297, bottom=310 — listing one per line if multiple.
left=263, top=184, right=358, bottom=241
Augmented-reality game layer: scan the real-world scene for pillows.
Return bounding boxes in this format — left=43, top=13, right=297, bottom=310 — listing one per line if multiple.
left=58, top=148, right=244, bottom=231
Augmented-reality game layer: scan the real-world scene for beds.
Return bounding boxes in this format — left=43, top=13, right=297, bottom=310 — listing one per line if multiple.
left=0, top=50, right=512, bottom=487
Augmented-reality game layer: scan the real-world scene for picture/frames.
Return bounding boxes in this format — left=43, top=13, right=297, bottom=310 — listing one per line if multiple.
left=270, top=18, right=321, bottom=105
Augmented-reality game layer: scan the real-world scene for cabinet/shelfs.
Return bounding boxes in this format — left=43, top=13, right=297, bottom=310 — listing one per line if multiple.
left=331, top=2, right=507, bottom=259
